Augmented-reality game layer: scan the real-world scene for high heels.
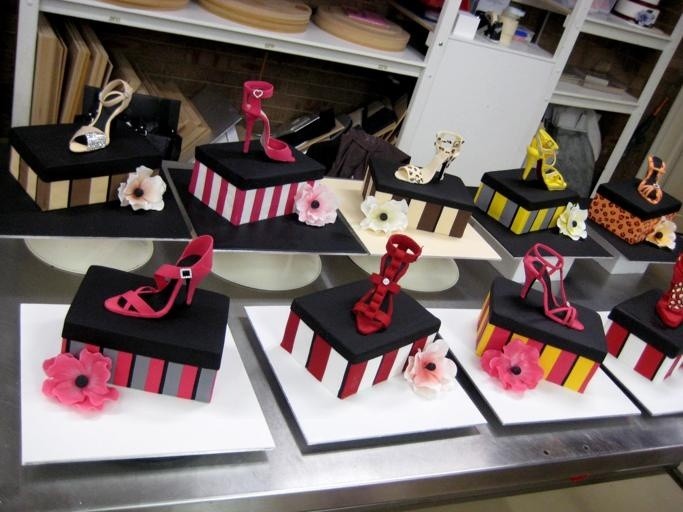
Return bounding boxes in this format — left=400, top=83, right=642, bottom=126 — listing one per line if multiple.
left=522, top=127, right=568, bottom=191
left=352, top=233, right=423, bottom=335
left=66, top=78, right=134, bottom=153
left=241, top=77, right=297, bottom=163
left=103, top=233, right=214, bottom=320
left=393, top=132, right=465, bottom=185
left=519, top=242, right=585, bottom=332
left=655, top=251, right=683, bottom=328
left=638, top=155, right=666, bottom=205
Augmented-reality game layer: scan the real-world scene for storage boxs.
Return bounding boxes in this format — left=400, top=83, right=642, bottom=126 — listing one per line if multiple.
left=280, top=276, right=442, bottom=401
left=187, top=140, right=326, bottom=226
left=588, top=180, right=682, bottom=243
left=9, top=123, right=163, bottom=210
left=363, top=163, right=472, bottom=239
left=476, top=276, right=608, bottom=393
left=474, top=167, right=581, bottom=234
left=60, top=264, right=230, bottom=401
left=605, top=289, right=682, bottom=383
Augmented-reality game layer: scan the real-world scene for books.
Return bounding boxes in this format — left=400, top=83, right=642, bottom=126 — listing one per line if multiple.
left=271, top=99, right=402, bottom=168
left=561, top=63, right=628, bottom=95
left=29, top=15, right=244, bottom=171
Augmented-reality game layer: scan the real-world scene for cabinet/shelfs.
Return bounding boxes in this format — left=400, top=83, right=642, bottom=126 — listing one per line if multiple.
left=16, top=1, right=682, bottom=201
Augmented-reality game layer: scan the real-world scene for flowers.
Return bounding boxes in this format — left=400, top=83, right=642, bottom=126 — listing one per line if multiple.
left=403, top=338, right=458, bottom=400
left=646, top=214, right=677, bottom=251
left=42, top=352, right=121, bottom=411
left=117, top=165, right=167, bottom=213
left=358, top=193, right=410, bottom=237
left=297, top=182, right=338, bottom=228
left=555, top=200, right=588, bottom=241
left=482, top=338, right=546, bottom=393
